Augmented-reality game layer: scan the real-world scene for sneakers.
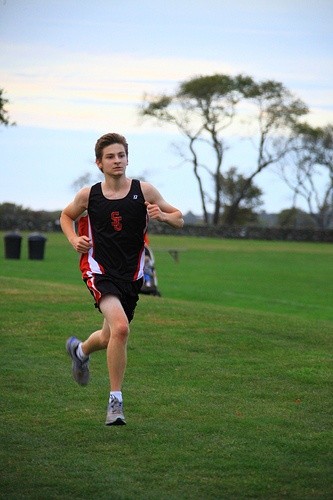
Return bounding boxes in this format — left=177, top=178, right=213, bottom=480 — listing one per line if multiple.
left=66, top=336, right=89, bottom=386
left=105, top=396, right=127, bottom=427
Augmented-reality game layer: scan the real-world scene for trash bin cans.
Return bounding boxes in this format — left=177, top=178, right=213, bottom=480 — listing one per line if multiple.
left=3, top=234, right=23, bottom=260
left=28, top=235, right=47, bottom=260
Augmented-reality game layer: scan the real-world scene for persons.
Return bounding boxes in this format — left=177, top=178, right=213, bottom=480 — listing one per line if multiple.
left=59, top=134, right=183, bottom=426
